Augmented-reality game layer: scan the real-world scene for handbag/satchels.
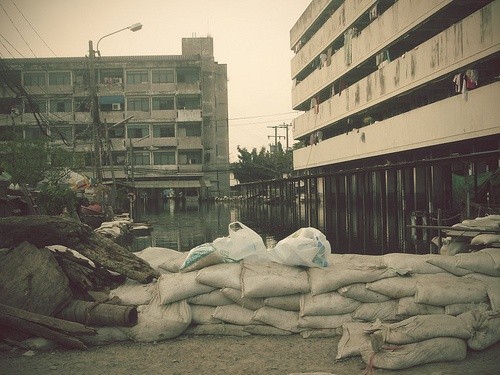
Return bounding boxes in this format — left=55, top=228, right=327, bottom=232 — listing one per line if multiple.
left=267, top=225, right=332, bottom=268
left=213, top=219, right=267, bottom=265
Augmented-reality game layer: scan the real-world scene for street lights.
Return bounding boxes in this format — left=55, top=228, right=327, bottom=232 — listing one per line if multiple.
left=96, top=23, right=143, bottom=50
left=133, top=135, right=150, bottom=144
left=108, top=114, right=135, bottom=131
